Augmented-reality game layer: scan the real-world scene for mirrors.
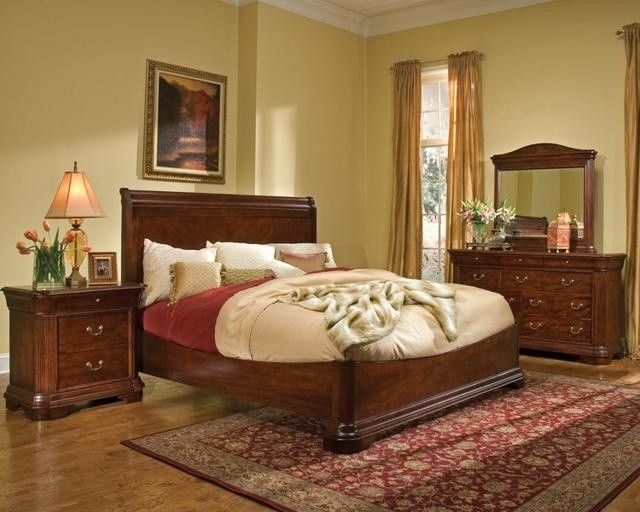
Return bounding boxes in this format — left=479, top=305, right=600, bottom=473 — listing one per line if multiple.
left=487, top=141, right=598, bottom=255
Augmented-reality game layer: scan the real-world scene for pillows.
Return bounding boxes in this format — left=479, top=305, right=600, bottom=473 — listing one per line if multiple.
left=135, top=233, right=339, bottom=314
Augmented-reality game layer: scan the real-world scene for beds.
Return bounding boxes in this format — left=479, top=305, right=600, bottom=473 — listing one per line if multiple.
left=113, top=182, right=527, bottom=457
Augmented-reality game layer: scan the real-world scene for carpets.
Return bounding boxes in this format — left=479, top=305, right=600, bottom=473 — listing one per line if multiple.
left=119, top=364, right=640, bottom=511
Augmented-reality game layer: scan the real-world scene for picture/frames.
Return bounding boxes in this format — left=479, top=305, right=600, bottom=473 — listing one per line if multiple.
left=140, top=56, right=231, bottom=188
left=84, top=250, right=119, bottom=287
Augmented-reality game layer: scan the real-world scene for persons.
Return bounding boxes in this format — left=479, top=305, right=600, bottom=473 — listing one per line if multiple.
left=97, top=261, right=109, bottom=275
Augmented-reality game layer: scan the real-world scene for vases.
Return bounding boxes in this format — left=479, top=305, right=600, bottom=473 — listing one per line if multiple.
left=492, top=218, right=507, bottom=241
left=464, top=219, right=491, bottom=246
left=29, top=247, right=66, bottom=293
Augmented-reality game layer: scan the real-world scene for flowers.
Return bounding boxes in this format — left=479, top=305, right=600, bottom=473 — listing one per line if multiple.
left=488, top=198, right=518, bottom=229
left=451, top=195, right=495, bottom=226
left=13, top=218, right=92, bottom=287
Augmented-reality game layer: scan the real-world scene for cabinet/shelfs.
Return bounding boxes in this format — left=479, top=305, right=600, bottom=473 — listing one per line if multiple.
left=440, top=243, right=630, bottom=366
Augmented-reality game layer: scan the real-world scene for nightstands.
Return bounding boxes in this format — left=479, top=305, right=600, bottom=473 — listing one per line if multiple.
left=1, top=274, right=149, bottom=423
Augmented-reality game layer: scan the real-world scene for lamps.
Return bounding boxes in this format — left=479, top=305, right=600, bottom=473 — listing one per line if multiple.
left=42, top=161, right=108, bottom=288
